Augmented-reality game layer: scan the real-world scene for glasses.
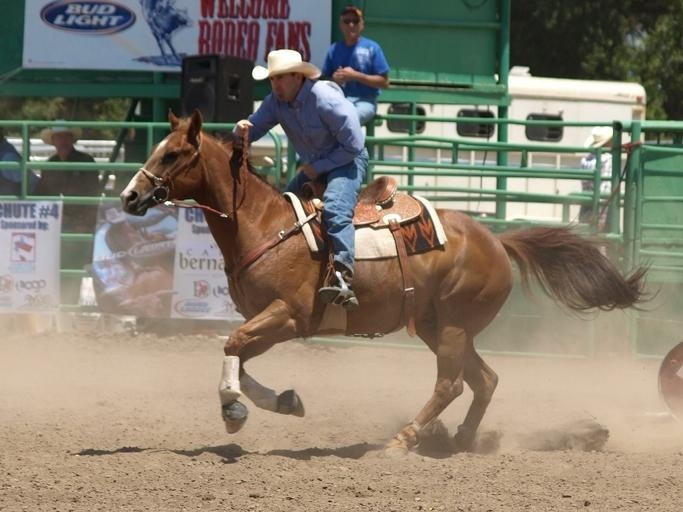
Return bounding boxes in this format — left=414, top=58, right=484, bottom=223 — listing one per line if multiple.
left=342, top=17, right=360, bottom=25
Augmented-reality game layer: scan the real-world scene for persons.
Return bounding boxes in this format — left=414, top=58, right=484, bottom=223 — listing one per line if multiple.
left=321, top=4, right=391, bottom=126
left=232, top=48, right=370, bottom=311
left=578, top=126, right=625, bottom=233
left=30, top=119, right=100, bottom=235
left=99, top=200, right=175, bottom=272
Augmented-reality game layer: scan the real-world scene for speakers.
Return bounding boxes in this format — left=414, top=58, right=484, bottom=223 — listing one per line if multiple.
left=180, top=53, right=254, bottom=124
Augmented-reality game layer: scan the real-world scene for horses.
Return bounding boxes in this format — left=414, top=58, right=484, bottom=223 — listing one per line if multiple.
left=119, top=108, right=661, bottom=457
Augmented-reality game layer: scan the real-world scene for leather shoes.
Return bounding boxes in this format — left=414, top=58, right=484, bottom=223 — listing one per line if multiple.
left=317, top=283, right=359, bottom=309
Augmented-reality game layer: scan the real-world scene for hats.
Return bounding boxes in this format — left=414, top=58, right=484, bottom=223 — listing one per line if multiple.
left=340, top=5, right=364, bottom=17
left=251, top=48, right=323, bottom=81
left=39, top=118, right=83, bottom=146
left=584, top=128, right=614, bottom=149
